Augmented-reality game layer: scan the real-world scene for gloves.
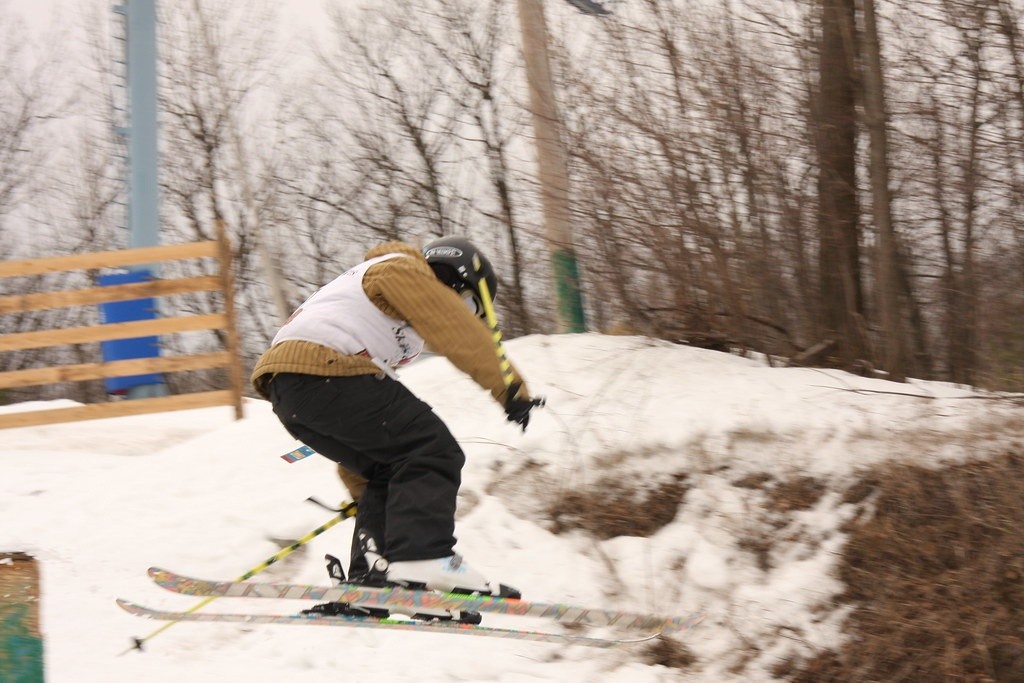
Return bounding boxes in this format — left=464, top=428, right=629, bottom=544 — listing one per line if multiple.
left=505, top=391, right=537, bottom=434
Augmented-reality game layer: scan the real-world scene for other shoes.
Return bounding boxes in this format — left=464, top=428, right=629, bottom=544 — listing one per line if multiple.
left=348, top=545, right=518, bottom=625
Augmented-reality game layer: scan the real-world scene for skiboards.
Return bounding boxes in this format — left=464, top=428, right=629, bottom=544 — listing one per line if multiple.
left=116, top=566, right=712, bottom=647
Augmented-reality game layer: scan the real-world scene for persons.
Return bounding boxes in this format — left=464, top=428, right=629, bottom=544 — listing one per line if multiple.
left=251, top=237, right=548, bottom=624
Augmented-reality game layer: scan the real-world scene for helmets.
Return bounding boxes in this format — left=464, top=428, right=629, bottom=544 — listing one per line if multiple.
left=428, top=231, right=505, bottom=304
